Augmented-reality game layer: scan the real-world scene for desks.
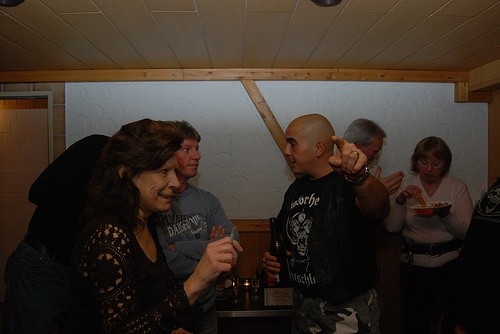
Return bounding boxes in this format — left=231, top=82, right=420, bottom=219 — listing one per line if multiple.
left=216, top=278, right=296, bottom=334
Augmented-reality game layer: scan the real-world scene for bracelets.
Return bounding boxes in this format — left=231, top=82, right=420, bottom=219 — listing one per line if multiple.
left=344, top=164, right=370, bottom=187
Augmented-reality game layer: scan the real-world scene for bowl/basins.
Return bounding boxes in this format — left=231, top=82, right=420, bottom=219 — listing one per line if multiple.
left=408, top=201, right=451, bottom=216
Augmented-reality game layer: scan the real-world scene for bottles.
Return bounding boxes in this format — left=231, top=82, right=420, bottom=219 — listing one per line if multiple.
left=254, top=270, right=263, bottom=305
left=266, top=218, right=293, bottom=286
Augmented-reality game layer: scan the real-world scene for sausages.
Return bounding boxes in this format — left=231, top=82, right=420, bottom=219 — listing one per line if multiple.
left=415, top=189, right=425, bottom=207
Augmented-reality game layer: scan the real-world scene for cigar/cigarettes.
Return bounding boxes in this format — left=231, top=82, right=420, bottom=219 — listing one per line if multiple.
left=230, top=226, right=238, bottom=244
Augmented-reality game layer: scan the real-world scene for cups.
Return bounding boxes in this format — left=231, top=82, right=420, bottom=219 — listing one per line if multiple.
left=208, top=222, right=240, bottom=257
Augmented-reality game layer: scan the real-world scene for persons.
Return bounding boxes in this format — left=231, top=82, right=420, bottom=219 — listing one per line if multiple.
left=3, top=119, right=243, bottom=334
left=262, top=114, right=500, bottom=334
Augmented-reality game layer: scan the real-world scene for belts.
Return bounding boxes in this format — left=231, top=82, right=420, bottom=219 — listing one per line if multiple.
left=304, top=287, right=334, bottom=298
